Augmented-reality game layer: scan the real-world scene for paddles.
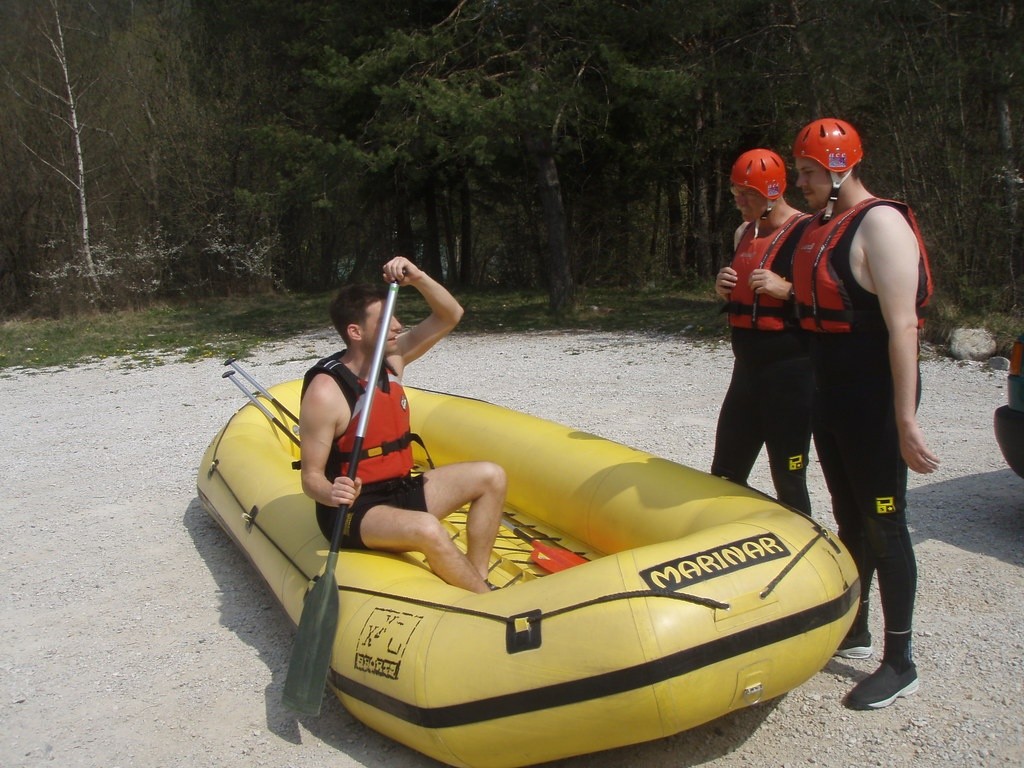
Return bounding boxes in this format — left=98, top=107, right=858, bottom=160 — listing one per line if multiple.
left=283, top=267, right=408, bottom=718
left=221, top=369, right=302, bottom=447
left=224, top=356, right=299, bottom=423
left=500, top=520, right=589, bottom=573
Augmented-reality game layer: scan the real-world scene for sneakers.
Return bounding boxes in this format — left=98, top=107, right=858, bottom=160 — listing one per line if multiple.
left=836, top=630, right=871, bottom=659
left=848, top=658, right=919, bottom=708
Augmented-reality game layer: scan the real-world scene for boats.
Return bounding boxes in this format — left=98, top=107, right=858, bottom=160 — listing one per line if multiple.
left=196, top=372, right=863, bottom=767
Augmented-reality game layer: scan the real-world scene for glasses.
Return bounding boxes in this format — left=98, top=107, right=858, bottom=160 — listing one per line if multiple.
left=730, top=185, right=760, bottom=197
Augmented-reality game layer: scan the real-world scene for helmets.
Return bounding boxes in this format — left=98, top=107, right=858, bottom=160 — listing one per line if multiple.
left=731, top=147, right=787, bottom=199
left=793, top=119, right=863, bottom=172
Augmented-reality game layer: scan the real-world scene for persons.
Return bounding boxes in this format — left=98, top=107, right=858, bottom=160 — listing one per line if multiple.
left=299, top=256, right=509, bottom=594
left=710, top=149, right=812, bottom=520
left=791, top=118, right=943, bottom=708
